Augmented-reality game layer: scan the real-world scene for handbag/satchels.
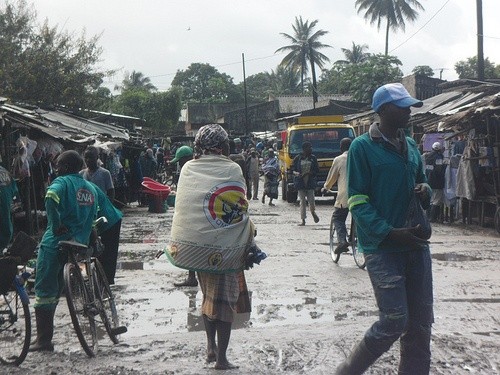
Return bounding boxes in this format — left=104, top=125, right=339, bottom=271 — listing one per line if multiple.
left=403, top=190, right=432, bottom=240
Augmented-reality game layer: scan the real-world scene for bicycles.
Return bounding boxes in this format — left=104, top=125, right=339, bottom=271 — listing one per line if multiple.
left=58, top=216, right=128, bottom=359
left=1, top=245, right=32, bottom=369
left=321, top=188, right=367, bottom=270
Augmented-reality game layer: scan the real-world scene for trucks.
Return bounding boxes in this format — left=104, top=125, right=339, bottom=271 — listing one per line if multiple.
left=276, top=114, right=357, bottom=205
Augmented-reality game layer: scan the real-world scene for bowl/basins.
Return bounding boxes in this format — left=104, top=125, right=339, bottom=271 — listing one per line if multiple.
left=141, top=181, right=170, bottom=199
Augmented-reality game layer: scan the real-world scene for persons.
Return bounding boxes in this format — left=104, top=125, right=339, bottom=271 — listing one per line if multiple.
left=422, top=142, right=446, bottom=224
left=165, top=124, right=267, bottom=370
left=78, top=146, right=114, bottom=204
left=26, top=149, right=99, bottom=353
left=0, top=154, right=18, bottom=258
left=131, top=129, right=281, bottom=207
left=334, top=82, right=435, bottom=375
left=320, top=137, right=353, bottom=254
left=84, top=179, right=123, bottom=290
left=291, top=141, right=320, bottom=227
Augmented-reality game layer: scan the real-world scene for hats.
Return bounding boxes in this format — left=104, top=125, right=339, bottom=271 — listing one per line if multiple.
left=250, top=148, right=256, bottom=153
left=371, top=83, right=423, bottom=112
left=171, top=146, right=193, bottom=163
left=432, top=142, right=442, bottom=150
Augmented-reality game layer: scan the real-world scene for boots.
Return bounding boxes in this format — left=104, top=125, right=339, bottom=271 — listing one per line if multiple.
left=336, top=338, right=378, bottom=375
left=397, top=341, right=431, bottom=375
left=27, top=304, right=54, bottom=352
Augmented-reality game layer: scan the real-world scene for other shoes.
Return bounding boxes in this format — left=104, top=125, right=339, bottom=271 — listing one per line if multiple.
left=173, top=279, right=197, bottom=287
left=348, top=237, right=353, bottom=244
left=335, top=242, right=348, bottom=254
